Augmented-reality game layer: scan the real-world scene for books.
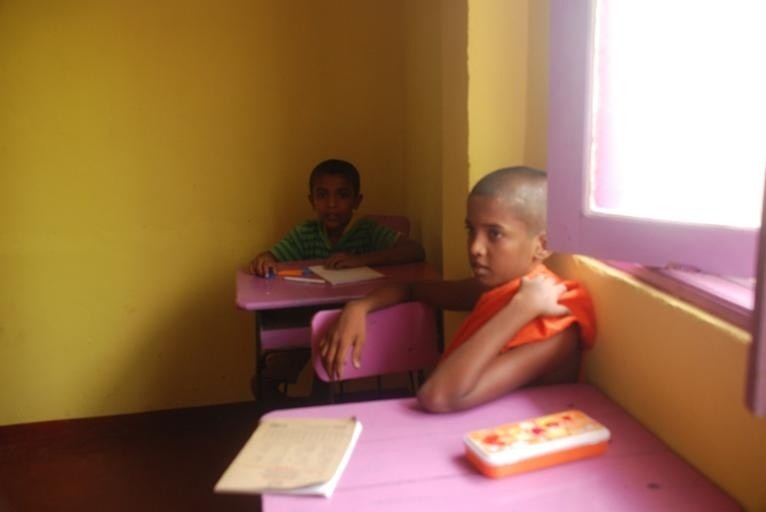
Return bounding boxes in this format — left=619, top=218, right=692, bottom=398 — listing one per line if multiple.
left=307, top=258, right=388, bottom=288
left=211, top=414, right=365, bottom=500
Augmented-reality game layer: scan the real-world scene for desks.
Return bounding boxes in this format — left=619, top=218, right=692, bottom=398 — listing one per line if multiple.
left=237, top=257, right=439, bottom=410
left=258, top=381, right=745, bottom=511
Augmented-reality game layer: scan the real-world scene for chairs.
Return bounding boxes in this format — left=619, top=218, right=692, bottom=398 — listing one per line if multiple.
left=287, top=214, right=410, bottom=401
left=309, top=304, right=439, bottom=397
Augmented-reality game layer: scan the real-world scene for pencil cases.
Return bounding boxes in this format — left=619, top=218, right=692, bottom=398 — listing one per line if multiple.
left=464, top=408, right=611, bottom=478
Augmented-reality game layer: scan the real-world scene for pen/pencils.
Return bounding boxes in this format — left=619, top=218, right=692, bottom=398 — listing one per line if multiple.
left=275, top=268, right=326, bottom=284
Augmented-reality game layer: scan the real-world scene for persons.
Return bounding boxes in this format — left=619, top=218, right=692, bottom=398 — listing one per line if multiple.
left=244, top=158, right=428, bottom=410
left=315, top=165, right=599, bottom=416
